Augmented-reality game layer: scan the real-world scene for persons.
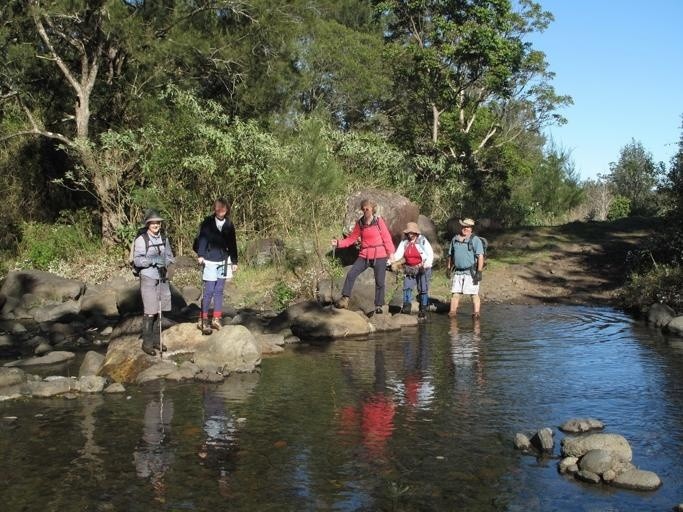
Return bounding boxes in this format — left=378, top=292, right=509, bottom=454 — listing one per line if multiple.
left=335, top=341, right=396, bottom=468
left=192, top=197, right=238, bottom=334
left=330, top=198, right=394, bottom=314
left=131, top=391, right=176, bottom=503
left=384, top=221, right=434, bottom=317
left=197, top=391, right=245, bottom=501
left=446, top=216, right=484, bottom=320
left=400, top=318, right=429, bottom=430
left=129, top=210, right=175, bottom=356
left=446, top=318, right=483, bottom=418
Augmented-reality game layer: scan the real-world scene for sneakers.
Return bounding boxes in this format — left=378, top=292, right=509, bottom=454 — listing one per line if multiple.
left=336, top=295, right=349, bottom=308
left=213, top=316, right=221, bottom=328
left=198, top=316, right=213, bottom=334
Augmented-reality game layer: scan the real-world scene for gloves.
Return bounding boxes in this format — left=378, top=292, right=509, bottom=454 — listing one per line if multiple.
left=446, top=269, right=452, bottom=278
left=473, top=271, right=482, bottom=281
left=153, top=256, right=169, bottom=268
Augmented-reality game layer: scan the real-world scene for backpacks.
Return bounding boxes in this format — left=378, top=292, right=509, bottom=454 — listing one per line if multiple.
left=193, top=214, right=214, bottom=258
left=129, top=227, right=165, bottom=279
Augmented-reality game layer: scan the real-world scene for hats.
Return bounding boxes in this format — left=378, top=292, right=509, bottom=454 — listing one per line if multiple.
left=459, top=218, right=475, bottom=227
left=402, top=222, right=420, bottom=233
left=144, top=208, right=165, bottom=226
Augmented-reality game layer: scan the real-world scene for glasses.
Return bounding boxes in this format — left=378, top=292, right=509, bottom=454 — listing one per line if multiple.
left=362, top=208, right=371, bottom=212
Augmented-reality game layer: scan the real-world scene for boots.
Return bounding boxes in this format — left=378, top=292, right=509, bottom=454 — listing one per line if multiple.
left=140, top=314, right=167, bottom=354
